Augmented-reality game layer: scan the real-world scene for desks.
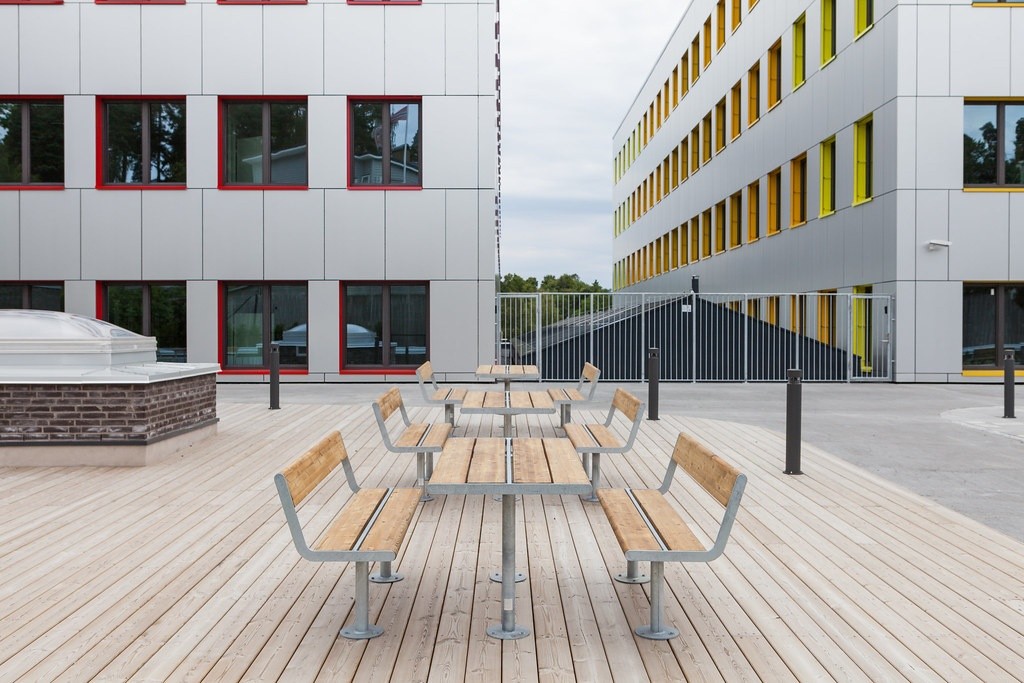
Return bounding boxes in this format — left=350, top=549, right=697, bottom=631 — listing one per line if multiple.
left=460, top=390, right=557, bottom=502
left=474, top=365, right=539, bottom=428
left=426, top=437, right=594, bottom=640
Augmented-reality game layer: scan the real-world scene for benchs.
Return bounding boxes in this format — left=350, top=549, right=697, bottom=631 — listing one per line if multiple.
left=596, top=432, right=747, bottom=641
left=372, top=385, right=451, bottom=504
left=547, top=362, right=601, bottom=439
left=417, top=361, right=468, bottom=438
left=275, top=431, right=425, bottom=639
left=562, top=387, right=647, bottom=502
